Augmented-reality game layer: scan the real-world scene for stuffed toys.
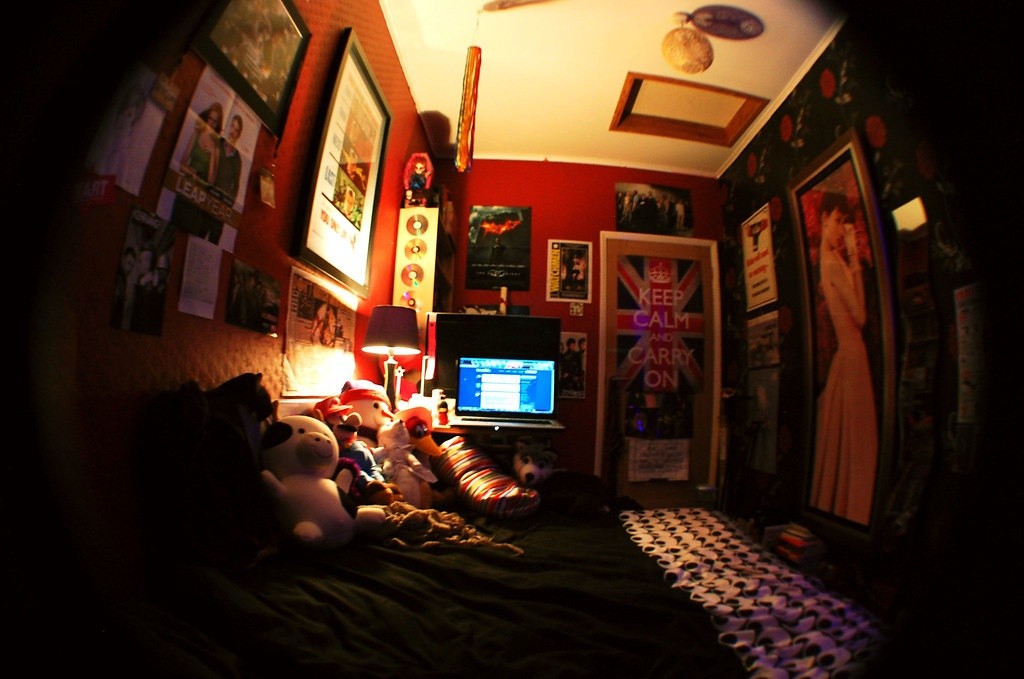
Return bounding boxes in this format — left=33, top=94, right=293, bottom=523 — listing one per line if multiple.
left=338, top=378, right=444, bottom=509
left=258, top=401, right=388, bottom=542
left=509, top=439, right=556, bottom=497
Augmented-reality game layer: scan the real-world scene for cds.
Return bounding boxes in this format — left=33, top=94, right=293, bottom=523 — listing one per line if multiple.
left=401, top=214, right=429, bottom=310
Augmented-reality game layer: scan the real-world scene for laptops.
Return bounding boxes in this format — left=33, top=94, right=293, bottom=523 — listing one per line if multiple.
left=447, top=356, right=565, bottom=429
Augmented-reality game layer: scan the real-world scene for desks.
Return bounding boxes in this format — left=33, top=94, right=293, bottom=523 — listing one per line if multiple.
left=402, top=406, right=565, bottom=488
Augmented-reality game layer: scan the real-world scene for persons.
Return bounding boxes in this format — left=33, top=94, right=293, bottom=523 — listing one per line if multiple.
left=216, top=112, right=245, bottom=205
left=116, top=247, right=168, bottom=333
left=232, top=266, right=279, bottom=331
left=180, top=101, right=222, bottom=192
left=812, top=193, right=875, bottom=524
left=616, top=188, right=687, bottom=232
left=557, top=337, right=584, bottom=390
left=310, top=396, right=394, bottom=504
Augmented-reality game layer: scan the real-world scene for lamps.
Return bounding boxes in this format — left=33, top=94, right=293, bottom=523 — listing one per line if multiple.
left=361, top=305, right=422, bottom=412
left=660, top=11, right=715, bottom=77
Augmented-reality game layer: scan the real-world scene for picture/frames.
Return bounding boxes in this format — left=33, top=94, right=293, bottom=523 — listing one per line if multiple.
left=740, top=202, right=778, bottom=312
left=193, top=0, right=312, bottom=141
left=784, top=122, right=898, bottom=538
left=294, top=26, right=394, bottom=299
